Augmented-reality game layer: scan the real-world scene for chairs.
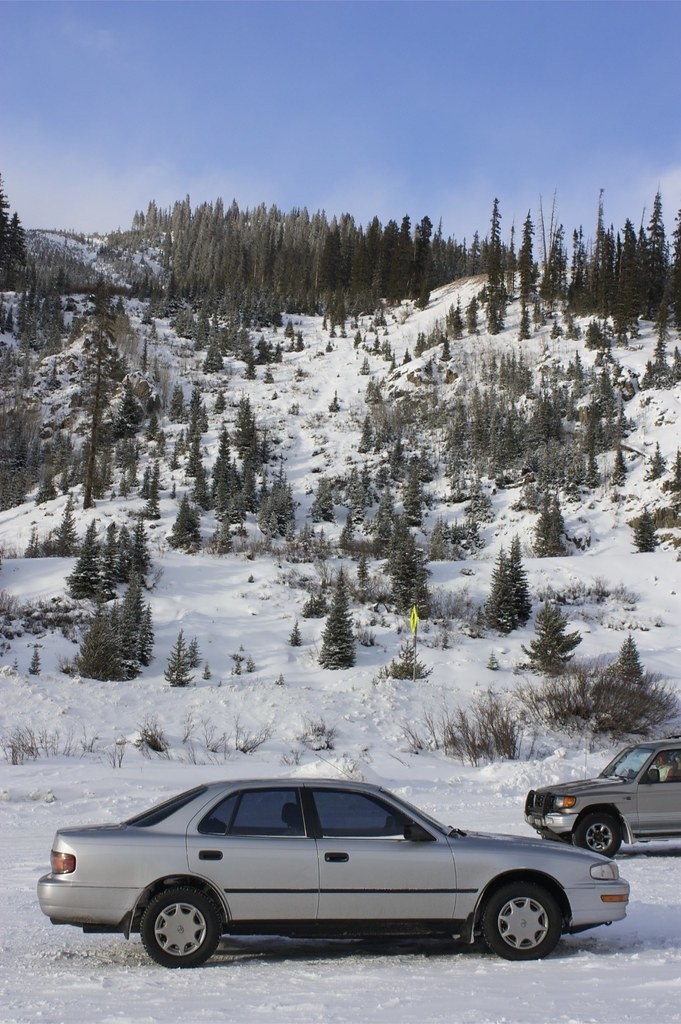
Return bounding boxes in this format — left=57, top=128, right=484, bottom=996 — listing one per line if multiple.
left=282, top=801, right=297, bottom=834
left=671, top=759, right=680, bottom=778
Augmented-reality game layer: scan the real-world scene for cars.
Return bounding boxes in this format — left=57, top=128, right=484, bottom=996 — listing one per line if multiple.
left=38, top=775, right=633, bottom=966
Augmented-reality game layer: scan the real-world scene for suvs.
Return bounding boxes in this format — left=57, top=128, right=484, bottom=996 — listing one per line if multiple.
left=527, top=735, right=680, bottom=861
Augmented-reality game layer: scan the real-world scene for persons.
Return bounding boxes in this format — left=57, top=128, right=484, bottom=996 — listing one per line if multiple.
left=647, top=751, right=674, bottom=781
